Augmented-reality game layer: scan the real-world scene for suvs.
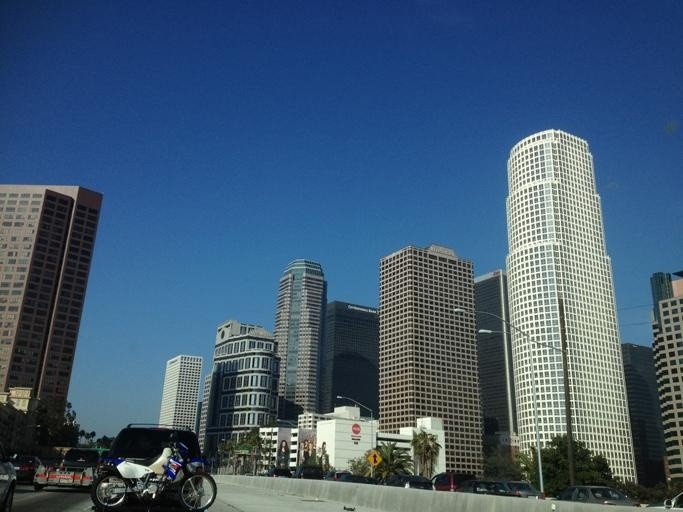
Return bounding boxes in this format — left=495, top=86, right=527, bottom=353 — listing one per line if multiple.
left=106, top=422, right=202, bottom=512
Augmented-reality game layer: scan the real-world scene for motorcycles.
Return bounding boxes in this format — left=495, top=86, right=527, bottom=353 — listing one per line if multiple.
left=89, top=431, right=217, bottom=512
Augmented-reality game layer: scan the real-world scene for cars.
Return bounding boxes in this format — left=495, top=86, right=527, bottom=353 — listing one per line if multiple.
left=60, top=448, right=103, bottom=473
left=0, top=454, right=46, bottom=512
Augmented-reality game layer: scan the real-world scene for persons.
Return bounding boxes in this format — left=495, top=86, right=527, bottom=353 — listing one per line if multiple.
left=277, top=440, right=290, bottom=469
left=297, top=440, right=316, bottom=467
left=319, top=442, right=329, bottom=473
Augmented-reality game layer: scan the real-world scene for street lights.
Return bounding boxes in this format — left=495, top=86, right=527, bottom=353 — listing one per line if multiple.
left=478, top=328, right=575, bottom=487
left=454, top=308, right=544, bottom=494
left=277, top=419, right=300, bottom=478
left=336, top=395, right=374, bottom=478
